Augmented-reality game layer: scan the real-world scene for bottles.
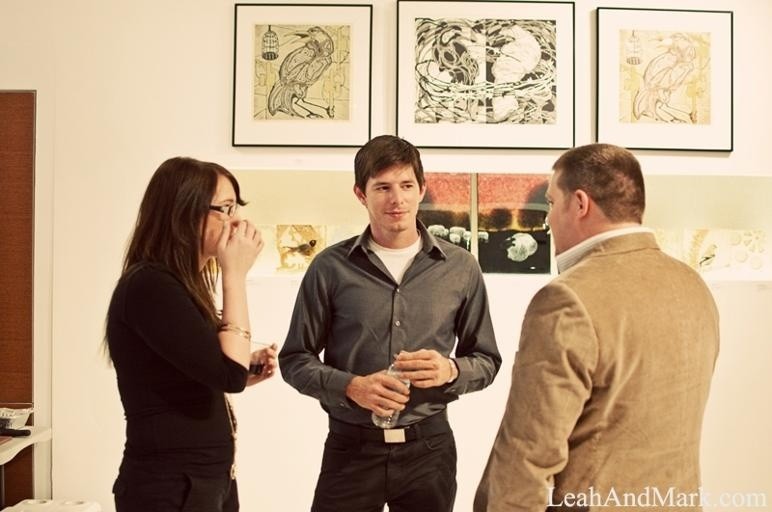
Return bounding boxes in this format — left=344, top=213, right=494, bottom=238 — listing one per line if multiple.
left=370, top=361, right=411, bottom=430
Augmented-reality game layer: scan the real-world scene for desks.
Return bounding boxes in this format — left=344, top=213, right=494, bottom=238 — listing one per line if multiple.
left=0, top=425, right=52, bottom=467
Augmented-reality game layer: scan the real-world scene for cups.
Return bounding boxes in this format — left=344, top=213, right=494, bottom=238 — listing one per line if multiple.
left=247, top=339, right=271, bottom=378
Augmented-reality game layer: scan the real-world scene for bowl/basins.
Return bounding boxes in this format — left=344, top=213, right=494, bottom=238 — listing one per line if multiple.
left=0, top=408, right=32, bottom=432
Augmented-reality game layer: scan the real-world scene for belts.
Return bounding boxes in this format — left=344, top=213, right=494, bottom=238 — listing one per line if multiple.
left=329, top=411, right=447, bottom=444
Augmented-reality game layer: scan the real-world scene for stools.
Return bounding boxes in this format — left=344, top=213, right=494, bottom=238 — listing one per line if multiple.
left=1, top=499, right=103, bottom=512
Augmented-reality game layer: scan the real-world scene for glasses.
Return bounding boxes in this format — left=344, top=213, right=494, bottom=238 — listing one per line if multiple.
left=209, top=204, right=239, bottom=218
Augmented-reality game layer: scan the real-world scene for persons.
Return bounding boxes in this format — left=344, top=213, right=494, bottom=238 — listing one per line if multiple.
left=97, top=156, right=277, bottom=510
left=472, top=143, right=720, bottom=511
left=278, top=135, right=501, bottom=511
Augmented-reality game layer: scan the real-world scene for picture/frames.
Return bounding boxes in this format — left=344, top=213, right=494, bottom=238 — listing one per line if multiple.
left=397, top=0, right=575, bottom=150
left=232, top=4, right=372, bottom=147
left=596, top=7, right=733, bottom=152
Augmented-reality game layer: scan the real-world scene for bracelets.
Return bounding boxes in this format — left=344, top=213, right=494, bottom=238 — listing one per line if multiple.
left=448, top=359, right=455, bottom=382
left=219, top=324, right=252, bottom=339
